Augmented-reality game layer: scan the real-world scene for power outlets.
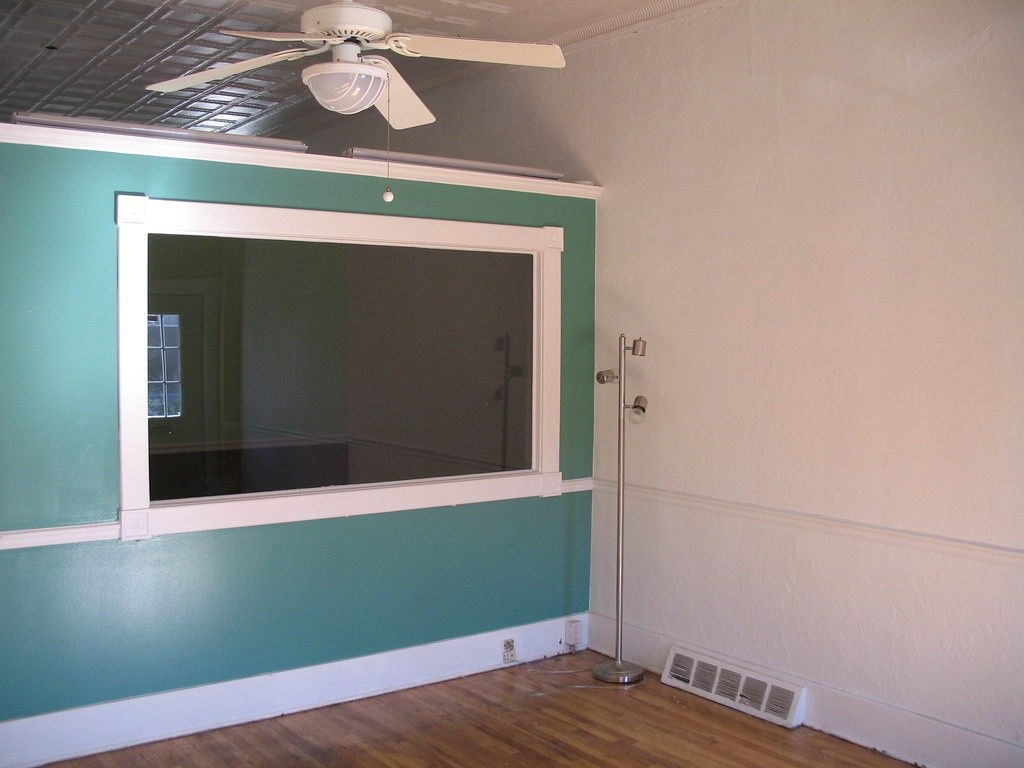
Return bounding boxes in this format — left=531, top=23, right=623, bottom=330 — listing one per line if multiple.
left=566, top=619, right=583, bottom=646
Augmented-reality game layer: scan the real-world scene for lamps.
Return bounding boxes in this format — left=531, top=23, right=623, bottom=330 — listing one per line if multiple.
left=342, top=146, right=566, bottom=180
left=10, top=109, right=309, bottom=154
left=301, top=42, right=395, bottom=203
left=596, top=333, right=650, bottom=684
left=491, top=333, right=522, bottom=474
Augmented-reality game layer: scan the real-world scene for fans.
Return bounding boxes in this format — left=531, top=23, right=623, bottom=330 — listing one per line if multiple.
left=144, top=0, right=569, bottom=131
